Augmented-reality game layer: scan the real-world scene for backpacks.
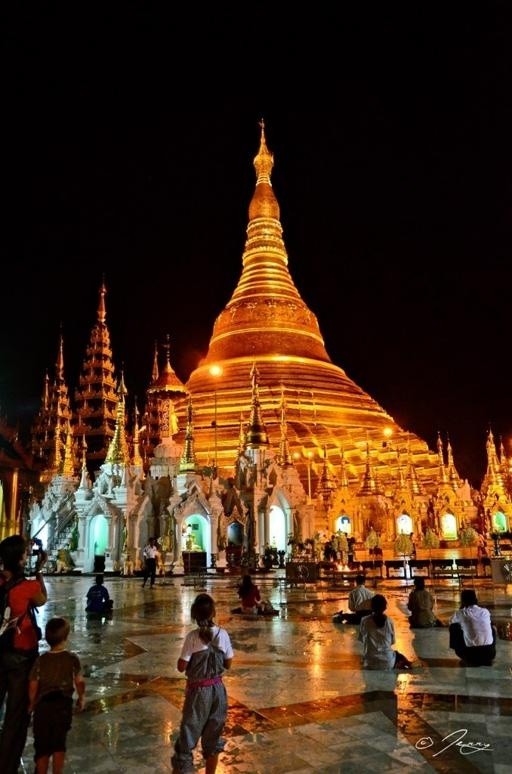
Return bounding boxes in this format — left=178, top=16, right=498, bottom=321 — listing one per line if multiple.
left=0, top=585, right=13, bottom=646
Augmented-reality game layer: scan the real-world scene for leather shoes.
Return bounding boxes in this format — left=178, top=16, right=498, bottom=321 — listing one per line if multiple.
left=460, top=659, right=470, bottom=666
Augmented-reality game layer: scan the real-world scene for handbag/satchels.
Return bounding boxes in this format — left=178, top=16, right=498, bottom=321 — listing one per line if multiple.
left=499, top=622, right=512, bottom=640
left=264, top=600, right=274, bottom=614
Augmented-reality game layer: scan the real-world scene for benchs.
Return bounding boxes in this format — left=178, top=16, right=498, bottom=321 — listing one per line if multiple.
left=431, top=568, right=476, bottom=583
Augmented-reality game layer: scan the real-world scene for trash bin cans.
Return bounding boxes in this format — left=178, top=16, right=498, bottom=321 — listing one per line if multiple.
left=94, top=555, right=105, bottom=571
left=492, top=559, right=505, bottom=583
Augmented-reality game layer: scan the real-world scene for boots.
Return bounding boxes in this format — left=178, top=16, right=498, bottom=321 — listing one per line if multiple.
left=396, top=653, right=412, bottom=670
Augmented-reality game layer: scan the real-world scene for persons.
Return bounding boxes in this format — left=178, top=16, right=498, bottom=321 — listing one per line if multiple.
left=169, top=593, right=233, bottom=774
left=1, top=531, right=48, bottom=773
left=355, top=593, right=410, bottom=669
left=141, top=536, right=158, bottom=590
left=448, top=587, right=495, bottom=666
left=29, top=618, right=87, bottom=773
left=331, top=575, right=375, bottom=623
left=235, top=574, right=265, bottom=615
left=83, top=575, right=115, bottom=615
left=405, top=576, right=447, bottom=628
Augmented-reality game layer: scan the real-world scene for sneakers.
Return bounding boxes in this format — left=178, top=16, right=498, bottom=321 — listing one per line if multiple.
left=439, top=622, right=446, bottom=626
